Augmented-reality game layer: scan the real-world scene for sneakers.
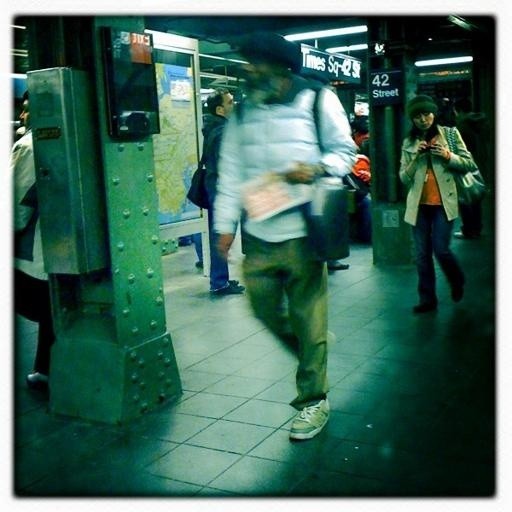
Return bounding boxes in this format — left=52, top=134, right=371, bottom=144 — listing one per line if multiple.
left=290, top=396, right=328, bottom=441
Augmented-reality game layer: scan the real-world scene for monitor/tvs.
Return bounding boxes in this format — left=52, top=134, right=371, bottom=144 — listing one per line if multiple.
left=99, top=26, right=160, bottom=139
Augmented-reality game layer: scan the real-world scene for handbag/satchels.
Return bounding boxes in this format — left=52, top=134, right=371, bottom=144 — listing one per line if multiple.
left=187, top=168, right=207, bottom=209
left=455, top=163, right=486, bottom=206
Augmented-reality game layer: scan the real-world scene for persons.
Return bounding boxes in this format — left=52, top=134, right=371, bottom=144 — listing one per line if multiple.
left=342, top=138, right=372, bottom=243
left=352, top=114, right=370, bottom=145
left=399, top=94, right=475, bottom=313
left=452, top=98, right=489, bottom=240
left=203, top=88, right=245, bottom=293
left=212, top=32, right=360, bottom=441
left=11, top=91, right=52, bottom=387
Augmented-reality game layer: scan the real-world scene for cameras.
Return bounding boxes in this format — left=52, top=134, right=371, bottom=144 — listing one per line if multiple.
left=418, top=145, right=437, bottom=154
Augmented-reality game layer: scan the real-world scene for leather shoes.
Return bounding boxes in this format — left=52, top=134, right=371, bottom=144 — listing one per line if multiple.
left=213, top=280, right=245, bottom=294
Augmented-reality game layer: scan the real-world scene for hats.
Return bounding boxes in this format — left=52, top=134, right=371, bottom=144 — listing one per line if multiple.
left=407, top=94, right=438, bottom=121
